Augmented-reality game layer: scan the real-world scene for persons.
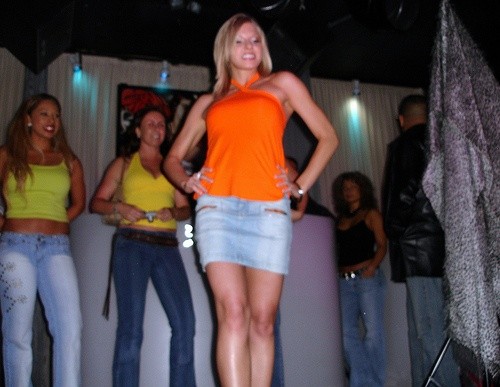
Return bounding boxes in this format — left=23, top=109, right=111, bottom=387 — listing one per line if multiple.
left=163, top=14, right=340, bottom=387
left=331, top=171, right=388, bottom=386
left=381, top=94, right=467, bottom=386
left=285, top=157, right=336, bottom=219
left=0, top=93, right=86, bottom=386
left=89, top=104, right=195, bottom=387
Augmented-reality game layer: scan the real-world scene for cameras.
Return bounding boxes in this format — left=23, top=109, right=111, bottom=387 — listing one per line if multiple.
left=144, top=211, right=158, bottom=222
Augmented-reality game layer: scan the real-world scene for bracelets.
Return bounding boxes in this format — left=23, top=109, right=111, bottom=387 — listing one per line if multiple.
left=113, top=200, right=122, bottom=226
left=293, top=181, right=306, bottom=201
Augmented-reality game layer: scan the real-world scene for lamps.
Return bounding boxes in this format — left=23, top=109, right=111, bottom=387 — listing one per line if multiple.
left=72, top=51, right=83, bottom=72
left=351, top=79, right=362, bottom=97
left=160, top=60, right=171, bottom=82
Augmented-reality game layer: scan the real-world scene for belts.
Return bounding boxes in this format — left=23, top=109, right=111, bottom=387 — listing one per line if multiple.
left=339, top=265, right=380, bottom=280
left=122, top=231, right=178, bottom=247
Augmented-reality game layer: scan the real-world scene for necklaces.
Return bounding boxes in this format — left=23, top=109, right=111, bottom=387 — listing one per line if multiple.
left=348, top=208, right=361, bottom=220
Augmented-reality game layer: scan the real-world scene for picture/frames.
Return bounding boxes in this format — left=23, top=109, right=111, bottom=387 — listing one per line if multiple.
left=117, top=83, right=213, bottom=177
